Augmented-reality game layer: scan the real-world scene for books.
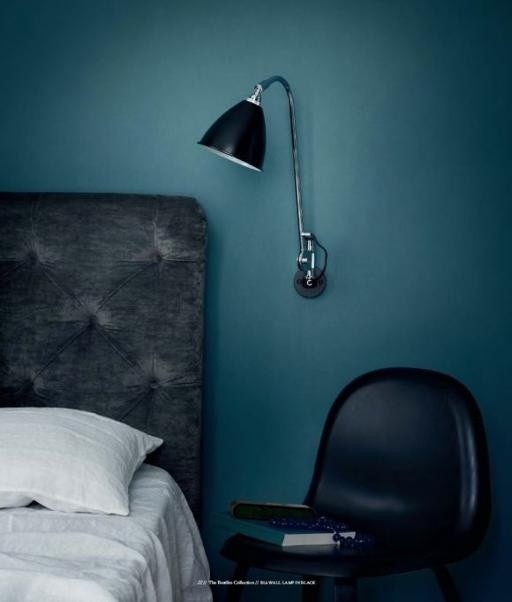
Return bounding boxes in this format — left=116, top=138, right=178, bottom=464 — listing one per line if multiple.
left=227, top=503, right=358, bottom=547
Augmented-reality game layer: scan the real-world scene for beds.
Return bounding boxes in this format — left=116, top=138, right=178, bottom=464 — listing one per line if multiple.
left=1, top=189, right=209, bottom=602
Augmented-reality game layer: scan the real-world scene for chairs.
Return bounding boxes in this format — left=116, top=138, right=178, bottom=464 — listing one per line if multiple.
left=221, top=367, right=491, bottom=602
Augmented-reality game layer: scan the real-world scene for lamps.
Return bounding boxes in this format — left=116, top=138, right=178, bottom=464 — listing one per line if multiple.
left=197, top=76, right=328, bottom=300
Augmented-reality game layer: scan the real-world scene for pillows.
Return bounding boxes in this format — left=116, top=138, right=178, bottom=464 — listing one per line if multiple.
left=0, top=406, right=163, bottom=516
